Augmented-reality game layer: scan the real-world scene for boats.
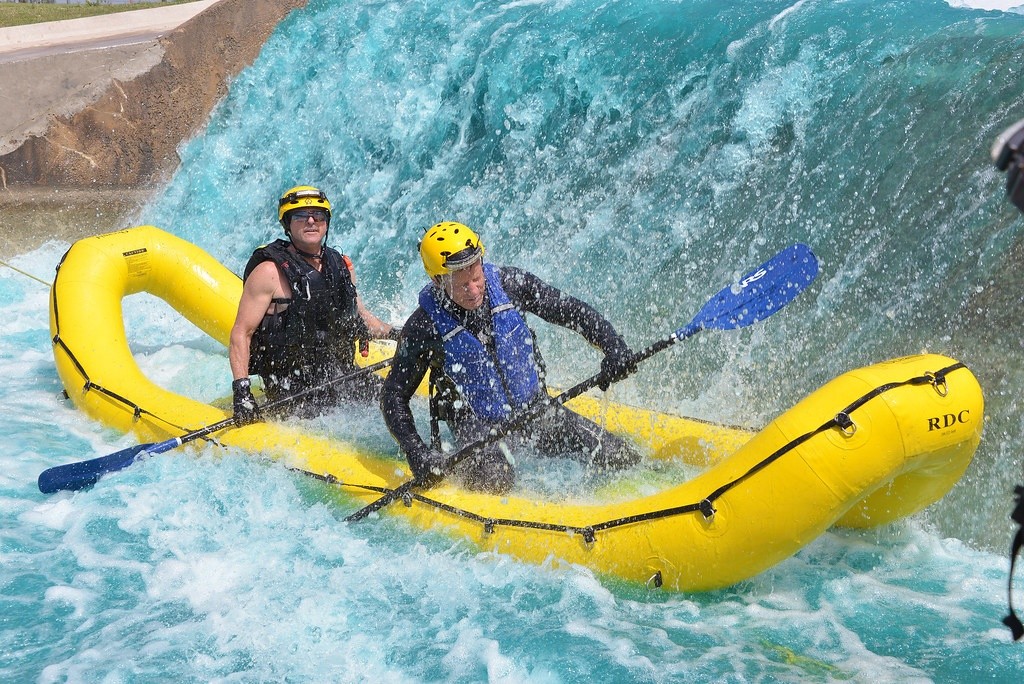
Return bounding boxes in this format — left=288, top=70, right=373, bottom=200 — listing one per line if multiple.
left=48, top=224, right=982, bottom=594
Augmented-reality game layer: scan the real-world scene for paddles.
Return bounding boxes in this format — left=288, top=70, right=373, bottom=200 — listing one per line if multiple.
left=37, top=354, right=399, bottom=496
left=342, top=243, right=822, bottom=524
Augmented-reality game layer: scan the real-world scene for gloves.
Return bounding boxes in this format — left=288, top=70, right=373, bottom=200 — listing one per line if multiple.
left=598, top=335, right=638, bottom=391
left=401, top=435, right=447, bottom=490
left=233, top=376, right=264, bottom=428
left=388, top=326, right=403, bottom=341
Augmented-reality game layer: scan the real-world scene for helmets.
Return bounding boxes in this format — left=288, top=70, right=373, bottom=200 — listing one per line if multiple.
left=420, top=222, right=484, bottom=284
left=280, top=186, right=331, bottom=226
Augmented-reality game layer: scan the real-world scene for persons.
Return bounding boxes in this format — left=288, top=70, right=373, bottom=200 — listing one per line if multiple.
left=229, top=186, right=401, bottom=428
left=380, top=221, right=637, bottom=495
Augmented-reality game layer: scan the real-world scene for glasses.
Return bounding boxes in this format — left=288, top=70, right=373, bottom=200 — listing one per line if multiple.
left=286, top=210, right=329, bottom=225
